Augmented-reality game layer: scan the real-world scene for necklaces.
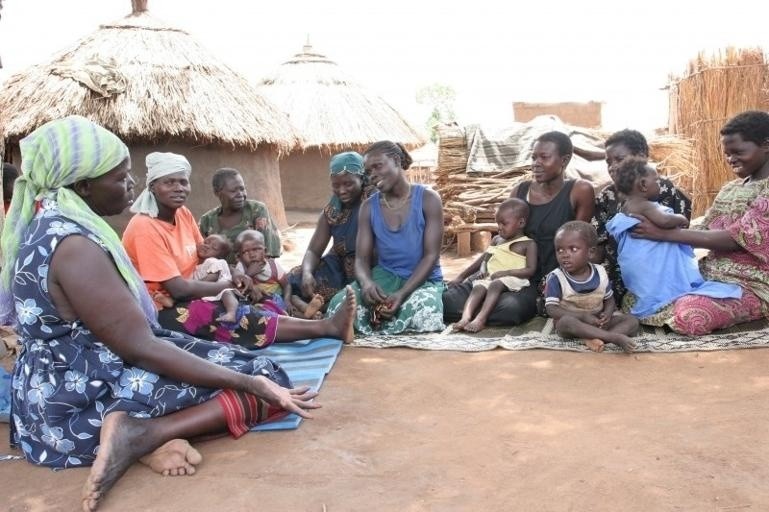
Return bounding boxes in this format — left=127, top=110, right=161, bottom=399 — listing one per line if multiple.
left=382, top=183, right=411, bottom=213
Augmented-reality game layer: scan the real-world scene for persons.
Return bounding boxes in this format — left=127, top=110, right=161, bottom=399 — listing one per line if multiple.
left=618, top=109, right=768, bottom=341
left=195, top=160, right=284, bottom=271
left=440, top=129, right=597, bottom=328
left=533, top=129, right=694, bottom=319
left=121, top=148, right=358, bottom=349
left=605, top=156, right=745, bottom=317
left=1, top=114, right=323, bottom=511
left=149, top=233, right=240, bottom=325
left=546, top=220, right=641, bottom=358
left=277, top=151, right=377, bottom=320
left=326, top=139, right=450, bottom=339
left=231, top=226, right=326, bottom=321
left=2, top=162, right=22, bottom=215
left=451, top=197, right=539, bottom=335
